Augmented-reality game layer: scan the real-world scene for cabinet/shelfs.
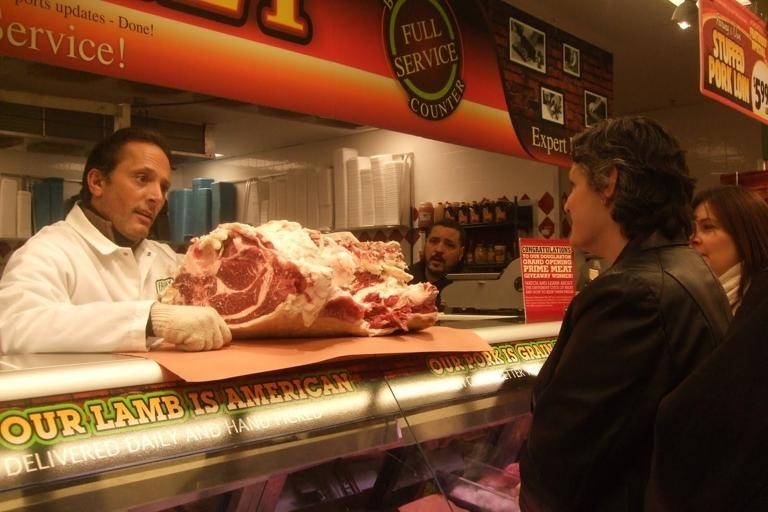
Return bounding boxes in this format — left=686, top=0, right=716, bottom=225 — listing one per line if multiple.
left=413, top=195, right=537, bottom=287
left=367, top=316, right=564, bottom=511
left=2, top=312, right=452, bottom=512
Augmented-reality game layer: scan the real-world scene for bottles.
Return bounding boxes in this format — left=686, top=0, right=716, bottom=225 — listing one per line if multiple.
left=418, top=196, right=516, bottom=266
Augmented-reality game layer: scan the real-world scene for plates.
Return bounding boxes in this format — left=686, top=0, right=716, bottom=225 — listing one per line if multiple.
left=1, top=171, right=65, bottom=240
left=148, top=177, right=236, bottom=243
left=249, top=147, right=411, bottom=228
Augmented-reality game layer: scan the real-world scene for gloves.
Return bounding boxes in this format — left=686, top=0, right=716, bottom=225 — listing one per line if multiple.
left=150, top=301, right=232, bottom=351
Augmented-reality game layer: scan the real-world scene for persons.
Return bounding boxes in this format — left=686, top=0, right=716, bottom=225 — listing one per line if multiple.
left=689, top=181, right=768, bottom=511
left=403, top=218, right=466, bottom=313
left=0, top=126, right=233, bottom=352
left=518, top=116, right=732, bottom=511
